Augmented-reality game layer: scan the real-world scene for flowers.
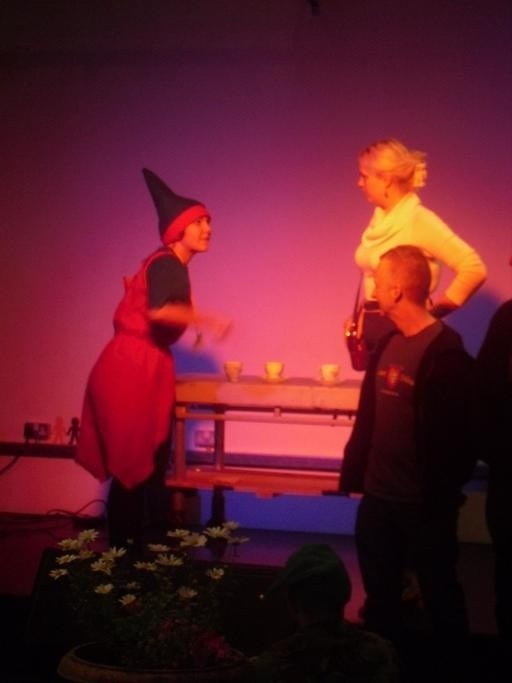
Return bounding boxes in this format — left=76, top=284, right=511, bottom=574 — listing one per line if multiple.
left=46, top=519, right=251, bottom=665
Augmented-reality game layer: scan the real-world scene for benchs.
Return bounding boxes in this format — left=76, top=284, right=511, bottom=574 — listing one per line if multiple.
left=167, top=469, right=342, bottom=501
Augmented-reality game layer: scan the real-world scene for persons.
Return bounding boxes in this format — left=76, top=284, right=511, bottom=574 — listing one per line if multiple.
left=72, top=167, right=232, bottom=563
left=322, top=137, right=488, bottom=497
left=339, top=245, right=469, bottom=638
left=471, top=299, right=512, bottom=683
left=236, top=542, right=402, bottom=682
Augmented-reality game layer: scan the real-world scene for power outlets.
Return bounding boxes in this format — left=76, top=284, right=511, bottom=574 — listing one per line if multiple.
left=24, top=420, right=51, bottom=439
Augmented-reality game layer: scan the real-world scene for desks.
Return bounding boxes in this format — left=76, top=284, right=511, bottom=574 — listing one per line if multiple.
left=164, top=375, right=365, bottom=542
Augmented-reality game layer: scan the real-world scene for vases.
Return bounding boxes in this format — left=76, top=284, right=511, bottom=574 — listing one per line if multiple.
left=57, top=638, right=247, bottom=683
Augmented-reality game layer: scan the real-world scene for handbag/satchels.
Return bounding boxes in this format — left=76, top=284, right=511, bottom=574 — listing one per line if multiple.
left=346, top=300, right=368, bottom=370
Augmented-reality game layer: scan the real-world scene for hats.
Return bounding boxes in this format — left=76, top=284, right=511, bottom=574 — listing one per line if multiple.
left=142, top=165, right=211, bottom=245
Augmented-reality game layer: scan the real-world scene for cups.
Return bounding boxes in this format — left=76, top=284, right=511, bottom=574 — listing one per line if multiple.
left=320, top=361, right=341, bottom=384
left=223, top=359, right=245, bottom=384
left=262, top=359, right=285, bottom=383
